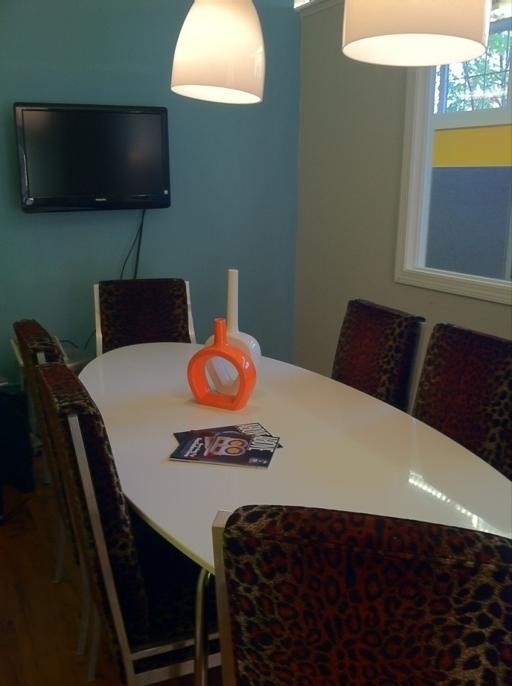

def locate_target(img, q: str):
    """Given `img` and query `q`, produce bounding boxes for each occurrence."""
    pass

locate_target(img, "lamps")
[339,0,489,66]
[171,0,267,106]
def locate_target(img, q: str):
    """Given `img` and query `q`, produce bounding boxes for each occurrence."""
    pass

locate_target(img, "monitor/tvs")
[13,100,172,214]
